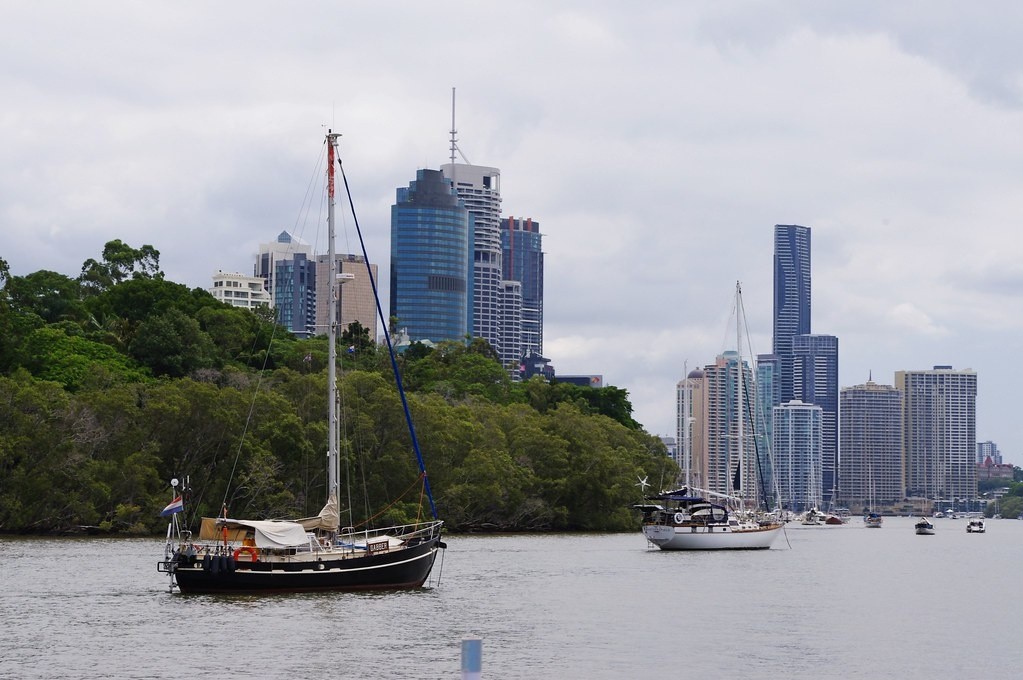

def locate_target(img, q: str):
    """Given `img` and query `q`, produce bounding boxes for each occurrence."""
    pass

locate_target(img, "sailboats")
[156,128,447,595]
[632,279,786,551]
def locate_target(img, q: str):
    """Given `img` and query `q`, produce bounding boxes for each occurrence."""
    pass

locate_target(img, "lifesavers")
[234,547,257,562]
[674,512,684,524]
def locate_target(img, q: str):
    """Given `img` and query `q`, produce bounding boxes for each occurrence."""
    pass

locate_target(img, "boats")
[801,485,851,525]
[966,519,986,533]
[914,517,936,535]
[864,511,883,528]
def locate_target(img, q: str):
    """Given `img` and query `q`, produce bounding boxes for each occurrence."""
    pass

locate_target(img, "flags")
[302,354,312,363]
[346,345,355,354]
[160,495,184,516]
